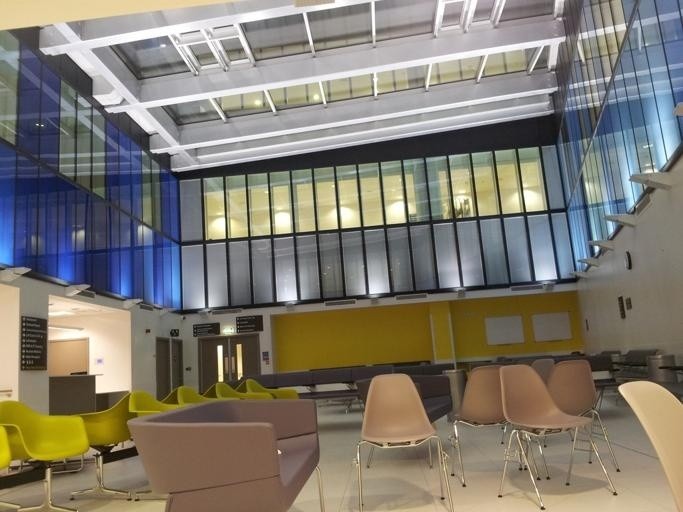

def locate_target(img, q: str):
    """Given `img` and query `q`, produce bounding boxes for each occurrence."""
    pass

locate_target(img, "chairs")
[497,363,620,510]
[349,371,457,512]
[0,399,92,509]
[449,364,529,487]
[70,392,135,503]
[0,347,683,511]
[542,357,620,487]
[125,398,327,511]
[611,376,683,510]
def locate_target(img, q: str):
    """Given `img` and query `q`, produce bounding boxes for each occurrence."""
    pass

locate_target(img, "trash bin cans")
[648,354,678,383]
